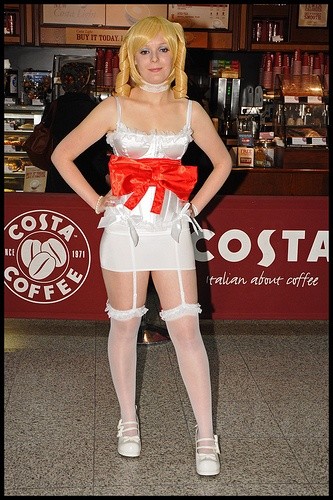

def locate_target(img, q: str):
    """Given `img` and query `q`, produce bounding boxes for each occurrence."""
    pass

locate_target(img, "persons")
[50,17,233,477]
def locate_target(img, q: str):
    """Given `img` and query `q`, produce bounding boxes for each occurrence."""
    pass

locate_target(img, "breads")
[4,124,34,173]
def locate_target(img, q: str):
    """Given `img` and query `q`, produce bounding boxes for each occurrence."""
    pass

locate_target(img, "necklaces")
[138,80,168,93]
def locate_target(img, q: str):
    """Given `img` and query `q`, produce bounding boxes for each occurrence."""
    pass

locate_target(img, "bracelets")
[192,205,199,217]
[95,195,103,215]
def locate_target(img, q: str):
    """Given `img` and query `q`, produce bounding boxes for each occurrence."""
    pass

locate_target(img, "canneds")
[6,13,17,34]
[254,20,280,42]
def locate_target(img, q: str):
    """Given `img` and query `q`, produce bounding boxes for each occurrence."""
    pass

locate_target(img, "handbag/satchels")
[23,100,58,171]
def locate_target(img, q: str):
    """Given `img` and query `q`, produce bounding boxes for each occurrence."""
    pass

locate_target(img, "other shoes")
[195,425,221,476]
[116,405,141,457]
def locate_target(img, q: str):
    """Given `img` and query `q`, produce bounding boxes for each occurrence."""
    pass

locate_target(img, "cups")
[260,49,329,96]
[287,118,294,125]
[296,118,303,125]
[95,49,119,86]
